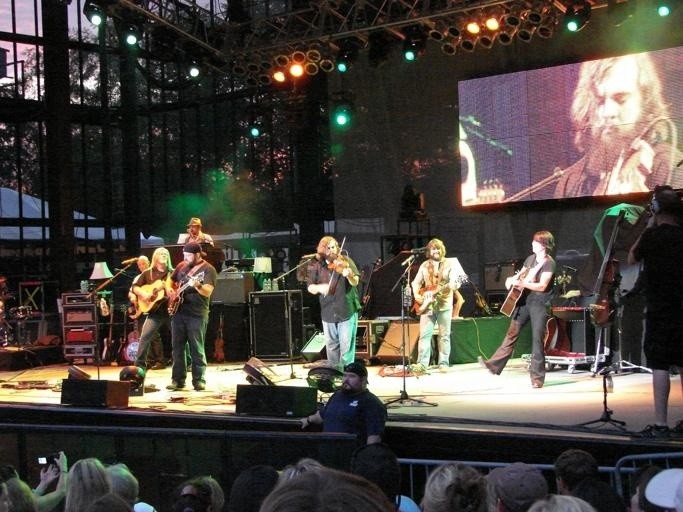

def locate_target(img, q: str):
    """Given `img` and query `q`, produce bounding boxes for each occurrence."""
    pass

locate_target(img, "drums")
[9,306,32,318]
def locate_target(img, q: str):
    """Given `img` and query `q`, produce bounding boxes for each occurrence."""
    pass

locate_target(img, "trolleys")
[541,305,606,373]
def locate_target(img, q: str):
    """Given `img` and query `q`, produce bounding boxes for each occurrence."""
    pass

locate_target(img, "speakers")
[234,382,317,421]
[207,270,254,304]
[58,379,129,409]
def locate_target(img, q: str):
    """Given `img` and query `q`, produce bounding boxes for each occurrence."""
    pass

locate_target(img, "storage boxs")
[18,281,58,314]
[250,292,303,359]
[355,320,390,359]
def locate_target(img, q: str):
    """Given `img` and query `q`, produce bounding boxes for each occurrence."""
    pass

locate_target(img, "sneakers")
[195,383,205,390]
[670,420,683,440]
[438,364,449,374]
[165,381,186,389]
[634,424,669,438]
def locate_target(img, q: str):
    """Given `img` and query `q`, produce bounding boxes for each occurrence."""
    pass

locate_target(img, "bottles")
[273,284,278,291]
[272,279,276,291]
[85,280,88,293]
[268,284,271,292]
[264,284,268,291]
[81,280,86,294]
[263,279,268,291]
[268,278,271,284]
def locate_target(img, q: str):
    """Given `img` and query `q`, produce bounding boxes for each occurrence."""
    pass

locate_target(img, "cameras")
[35,453,61,468]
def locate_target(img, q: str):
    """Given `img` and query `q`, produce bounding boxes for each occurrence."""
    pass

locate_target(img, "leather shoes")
[477,355,495,375]
[532,381,543,388]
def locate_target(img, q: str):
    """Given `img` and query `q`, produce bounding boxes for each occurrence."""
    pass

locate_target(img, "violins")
[592,138,655,198]
[328,254,357,285]
[213,315,225,362]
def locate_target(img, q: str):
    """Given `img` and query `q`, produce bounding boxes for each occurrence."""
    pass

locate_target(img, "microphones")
[121,258,139,265]
[301,254,319,260]
[586,121,620,183]
[410,247,429,254]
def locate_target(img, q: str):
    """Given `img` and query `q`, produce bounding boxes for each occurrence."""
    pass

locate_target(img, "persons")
[129,247,191,372]
[261,473,398,512]
[350,444,421,512]
[184,218,212,245]
[128,255,165,369]
[411,239,468,377]
[66,458,111,512]
[298,363,392,472]
[476,231,559,389]
[626,185,683,438]
[307,237,360,373]
[0,451,67,512]
[549,51,683,198]
[164,243,217,391]
[422,460,488,511]
[554,449,598,496]
[169,476,211,511]
[106,463,155,512]
[630,466,683,512]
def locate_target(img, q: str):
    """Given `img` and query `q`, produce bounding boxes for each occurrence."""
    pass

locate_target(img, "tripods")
[383,256,440,407]
[268,260,308,384]
[576,325,631,431]
[401,255,415,266]
[384,262,431,380]
[598,269,652,375]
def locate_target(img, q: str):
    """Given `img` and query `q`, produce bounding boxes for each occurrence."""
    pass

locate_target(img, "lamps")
[253,256,272,289]
[89,262,114,280]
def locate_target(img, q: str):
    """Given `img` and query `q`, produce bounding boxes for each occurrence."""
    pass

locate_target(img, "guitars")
[125,314,140,361]
[544,318,571,356]
[361,257,381,315]
[168,271,204,315]
[410,274,468,315]
[102,304,117,361]
[118,304,131,363]
[500,267,530,317]
[138,280,168,314]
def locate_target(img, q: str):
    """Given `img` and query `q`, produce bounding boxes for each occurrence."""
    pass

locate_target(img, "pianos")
[141,242,226,274]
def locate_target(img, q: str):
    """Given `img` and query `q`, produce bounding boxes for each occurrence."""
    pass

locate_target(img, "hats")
[350,442,401,496]
[645,467,683,512]
[182,242,208,258]
[186,217,203,228]
[343,362,369,385]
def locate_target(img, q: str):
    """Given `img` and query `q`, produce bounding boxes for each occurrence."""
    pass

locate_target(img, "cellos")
[589,210,625,325]
